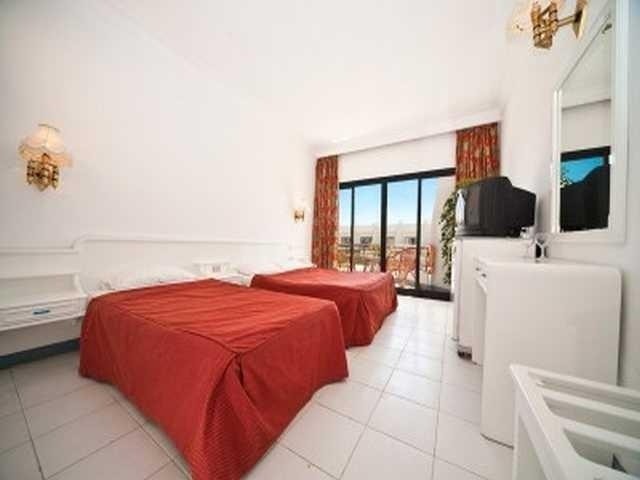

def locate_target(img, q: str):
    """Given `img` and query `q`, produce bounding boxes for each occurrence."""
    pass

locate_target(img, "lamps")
[508,0,591,51]
[15,120,74,192]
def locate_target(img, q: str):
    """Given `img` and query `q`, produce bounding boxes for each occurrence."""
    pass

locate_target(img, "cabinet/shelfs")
[450,235,537,358]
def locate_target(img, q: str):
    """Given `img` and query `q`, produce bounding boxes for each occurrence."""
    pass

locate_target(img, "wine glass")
[534,233,550,262]
[520,226,536,262]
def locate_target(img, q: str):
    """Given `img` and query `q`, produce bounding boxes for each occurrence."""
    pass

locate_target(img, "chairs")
[337,235,434,274]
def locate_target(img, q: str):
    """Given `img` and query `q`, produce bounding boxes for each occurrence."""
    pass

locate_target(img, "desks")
[469,246,623,448]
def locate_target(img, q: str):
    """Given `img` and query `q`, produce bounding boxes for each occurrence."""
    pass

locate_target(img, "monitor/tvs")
[560,164,611,231]
[465,175,536,237]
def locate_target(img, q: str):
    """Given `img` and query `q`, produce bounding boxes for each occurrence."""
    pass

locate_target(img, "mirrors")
[554,10,615,232]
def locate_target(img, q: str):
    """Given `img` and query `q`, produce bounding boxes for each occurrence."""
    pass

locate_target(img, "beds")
[78,278,350,480]
[251,266,399,346]
